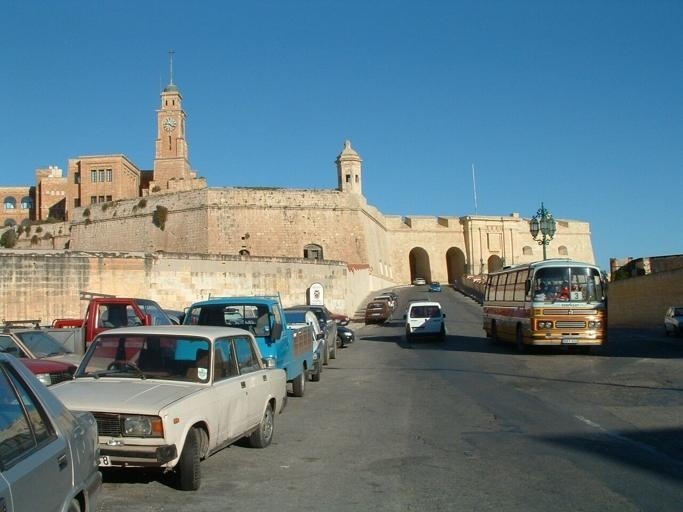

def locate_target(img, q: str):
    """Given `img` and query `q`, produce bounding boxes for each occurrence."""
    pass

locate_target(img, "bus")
[481,257,609,356]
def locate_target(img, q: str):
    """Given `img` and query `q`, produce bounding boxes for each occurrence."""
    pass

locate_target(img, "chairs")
[136,347,226,381]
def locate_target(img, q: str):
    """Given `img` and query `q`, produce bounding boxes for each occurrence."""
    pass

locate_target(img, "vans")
[402,301,446,343]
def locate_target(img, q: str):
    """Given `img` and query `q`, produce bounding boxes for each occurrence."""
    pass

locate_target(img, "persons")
[534,282,582,300]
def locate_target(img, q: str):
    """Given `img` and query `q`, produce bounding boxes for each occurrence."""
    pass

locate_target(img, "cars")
[364,292,399,325]
[413,277,426,285]
[664,306,683,334]
[429,281,442,293]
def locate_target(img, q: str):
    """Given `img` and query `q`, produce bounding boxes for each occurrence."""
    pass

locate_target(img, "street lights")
[528,202,557,261]
